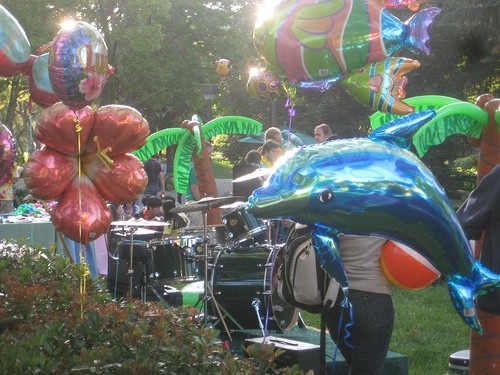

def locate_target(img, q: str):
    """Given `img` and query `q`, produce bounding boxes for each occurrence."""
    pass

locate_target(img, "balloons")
[0,123,17,178]
[246,136,500,336]
[0,5,115,108]
[47,22,109,111]
[23,100,150,243]
[215,0,442,115]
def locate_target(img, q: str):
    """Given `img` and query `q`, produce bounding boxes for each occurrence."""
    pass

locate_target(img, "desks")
[0,218,108,275]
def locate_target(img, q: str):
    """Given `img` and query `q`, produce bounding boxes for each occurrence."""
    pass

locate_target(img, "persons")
[314,124,337,144]
[454,162,500,375]
[322,234,394,375]
[142,155,167,204]
[106,195,205,230]
[12,162,27,210]
[233,127,284,196]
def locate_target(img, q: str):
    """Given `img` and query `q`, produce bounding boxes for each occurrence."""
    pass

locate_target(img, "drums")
[206,245,299,333]
[220,203,267,244]
[116,224,228,287]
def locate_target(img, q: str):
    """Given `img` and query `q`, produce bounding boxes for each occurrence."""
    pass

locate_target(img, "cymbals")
[109,218,172,227]
[109,227,161,236]
[169,195,248,214]
[218,201,245,209]
